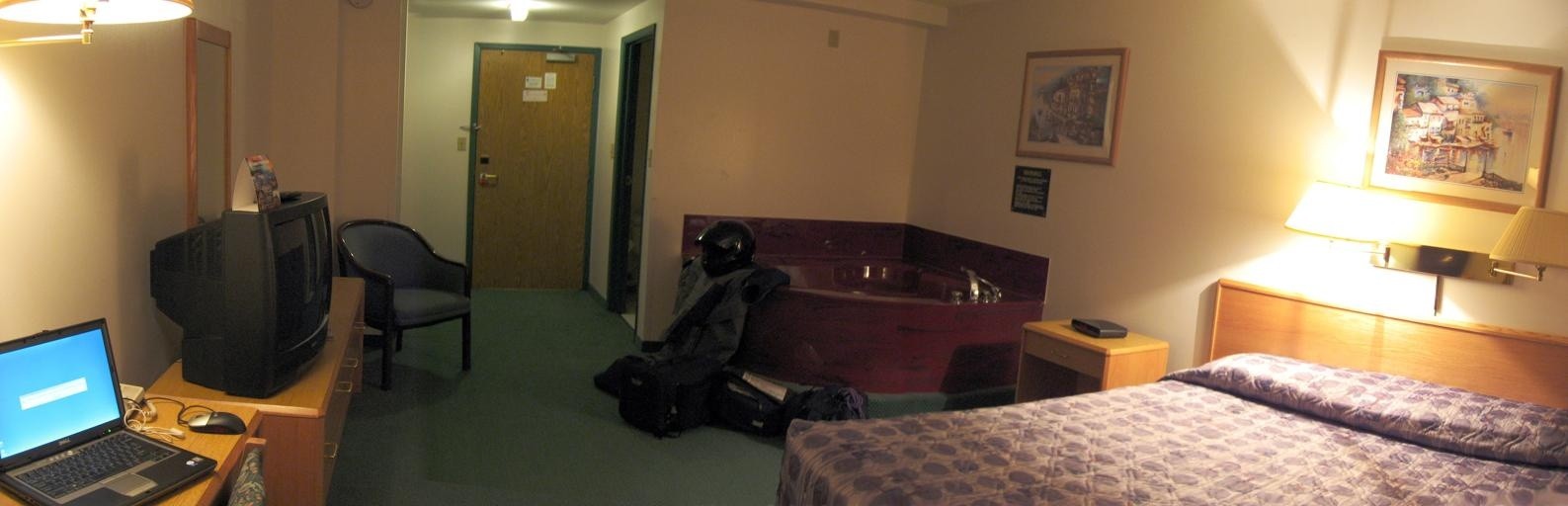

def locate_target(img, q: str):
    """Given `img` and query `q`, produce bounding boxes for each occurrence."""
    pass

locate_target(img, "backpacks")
[715,368,797,437]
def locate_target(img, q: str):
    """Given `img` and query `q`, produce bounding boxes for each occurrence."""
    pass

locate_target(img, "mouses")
[190,412,246,434]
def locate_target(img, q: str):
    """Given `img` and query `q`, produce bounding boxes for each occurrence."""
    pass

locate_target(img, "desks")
[1013,319,1170,403]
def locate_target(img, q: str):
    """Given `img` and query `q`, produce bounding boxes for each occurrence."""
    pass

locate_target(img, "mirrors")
[184,17,234,229]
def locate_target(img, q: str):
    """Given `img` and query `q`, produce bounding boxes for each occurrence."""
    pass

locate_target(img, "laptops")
[0,317,217,506]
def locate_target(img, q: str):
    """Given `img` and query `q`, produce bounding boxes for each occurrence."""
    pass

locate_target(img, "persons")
[592,217,788,438]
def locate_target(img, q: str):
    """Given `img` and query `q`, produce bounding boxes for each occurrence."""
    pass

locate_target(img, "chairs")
[336,219,473,391]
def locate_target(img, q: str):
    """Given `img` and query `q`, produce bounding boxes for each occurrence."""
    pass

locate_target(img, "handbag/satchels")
[779,372,868,437]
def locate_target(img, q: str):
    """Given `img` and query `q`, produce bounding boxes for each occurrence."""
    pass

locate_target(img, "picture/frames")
[1361,49,1563,216]
[1014,46,1130,167]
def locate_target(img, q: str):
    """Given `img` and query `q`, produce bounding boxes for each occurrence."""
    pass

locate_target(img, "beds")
[775,279,1568,506]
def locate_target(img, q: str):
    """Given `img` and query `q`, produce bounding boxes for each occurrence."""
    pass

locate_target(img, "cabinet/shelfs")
[144,277,366,506]
[1,398,265,506]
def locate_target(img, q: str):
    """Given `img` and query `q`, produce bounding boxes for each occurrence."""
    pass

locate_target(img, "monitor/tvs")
[159,191,333,398]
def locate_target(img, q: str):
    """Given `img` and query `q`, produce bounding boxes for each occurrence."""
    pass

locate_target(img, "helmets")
[694,220,754,267]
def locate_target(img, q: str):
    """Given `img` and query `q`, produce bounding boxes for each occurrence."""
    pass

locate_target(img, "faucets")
[979,278,1001,301]
[960,265,980,301]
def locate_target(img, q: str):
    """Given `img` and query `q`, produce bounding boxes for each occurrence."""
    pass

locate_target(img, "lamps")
[0,0,194,47]
[1488,207,1568,281]
[1284,179,1385,245]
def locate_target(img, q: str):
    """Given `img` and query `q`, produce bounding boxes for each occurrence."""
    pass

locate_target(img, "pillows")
[1164,353,1568,467]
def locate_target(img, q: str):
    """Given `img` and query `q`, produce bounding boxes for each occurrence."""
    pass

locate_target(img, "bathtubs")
[758,253,1045,391]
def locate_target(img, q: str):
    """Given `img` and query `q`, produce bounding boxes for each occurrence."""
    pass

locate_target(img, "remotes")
[280,191,301,201]
